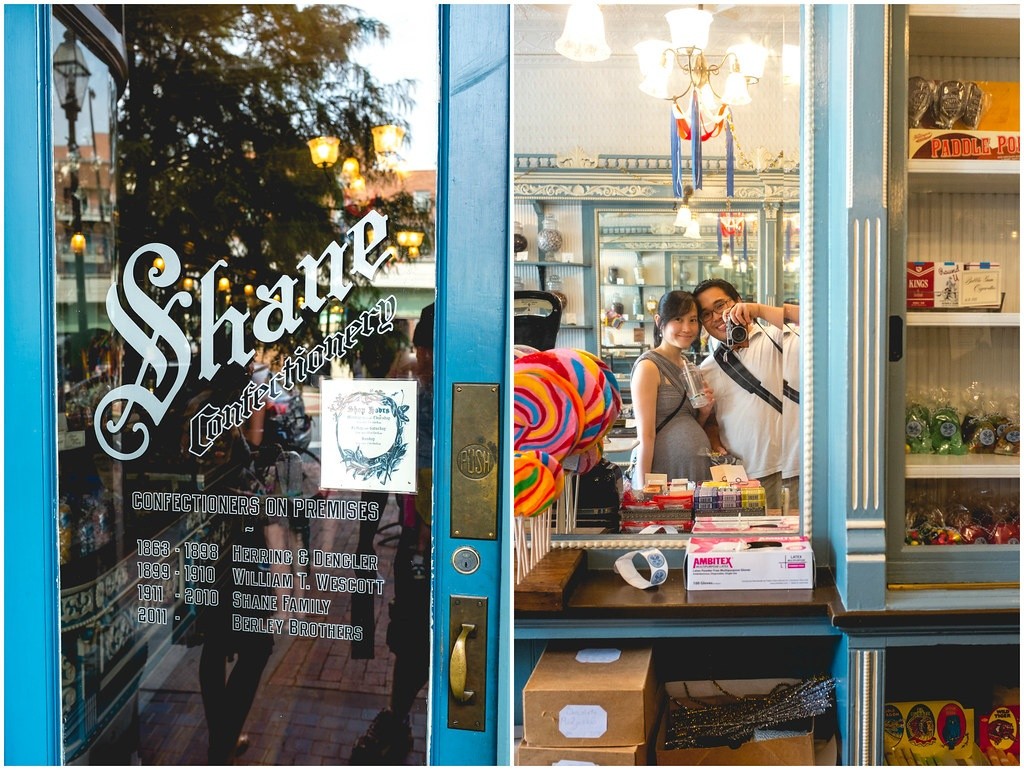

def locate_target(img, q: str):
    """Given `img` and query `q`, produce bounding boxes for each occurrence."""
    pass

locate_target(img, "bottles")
[252,362,288,402]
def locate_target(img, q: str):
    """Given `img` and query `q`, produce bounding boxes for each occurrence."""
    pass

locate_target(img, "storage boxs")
[681,534,817,591]
[617,479,800,532]
[906,126,1020,162]
[515,641,839,766]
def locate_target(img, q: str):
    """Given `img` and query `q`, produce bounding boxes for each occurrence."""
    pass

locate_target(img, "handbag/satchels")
[351,545,376,659]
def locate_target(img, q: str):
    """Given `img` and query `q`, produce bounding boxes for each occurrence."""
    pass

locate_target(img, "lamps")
[303,122,424,263]
[555,4,613,62]
[718,243,747,274]
[67,230,258,299]
[632,4,771,125]
[673,186,703,240]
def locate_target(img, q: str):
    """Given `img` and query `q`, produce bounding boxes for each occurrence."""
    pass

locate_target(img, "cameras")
[726,316,749,349]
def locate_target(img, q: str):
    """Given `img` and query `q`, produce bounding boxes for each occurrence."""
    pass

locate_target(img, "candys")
[512,348,625,523]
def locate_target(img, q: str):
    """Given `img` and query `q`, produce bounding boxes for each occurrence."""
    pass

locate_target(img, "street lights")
[51,29,95,452]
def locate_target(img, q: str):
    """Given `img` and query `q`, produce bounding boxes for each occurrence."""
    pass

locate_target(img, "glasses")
[698,297,734,324]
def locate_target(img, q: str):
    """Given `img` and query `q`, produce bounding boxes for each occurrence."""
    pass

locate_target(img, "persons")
[628,290,715,492]
[783,301,802,510]
[694,277,783,510]
[198,302,437,767]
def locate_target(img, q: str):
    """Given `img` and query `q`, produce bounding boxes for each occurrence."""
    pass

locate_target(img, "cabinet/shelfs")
[513,260,595,329]
[885,4,1020,586]
[600,282,698,323]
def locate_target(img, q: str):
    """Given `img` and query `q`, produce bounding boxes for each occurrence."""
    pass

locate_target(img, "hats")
[412,304,434,344]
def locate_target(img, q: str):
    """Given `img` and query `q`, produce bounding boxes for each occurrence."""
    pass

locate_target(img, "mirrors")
[593,208,801,393]
[510,4,817,554]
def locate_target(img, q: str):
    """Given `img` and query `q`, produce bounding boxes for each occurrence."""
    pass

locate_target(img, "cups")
[678,363,707,409]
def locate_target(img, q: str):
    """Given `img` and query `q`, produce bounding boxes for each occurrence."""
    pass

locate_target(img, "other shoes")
[209,734,260,766]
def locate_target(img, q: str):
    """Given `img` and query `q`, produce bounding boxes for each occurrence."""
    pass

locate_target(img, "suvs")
[148,361,313,460]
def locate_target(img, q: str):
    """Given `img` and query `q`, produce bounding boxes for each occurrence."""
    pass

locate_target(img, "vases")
[513,221,528,262]
[546,274,568,327]
[536,214,563,262]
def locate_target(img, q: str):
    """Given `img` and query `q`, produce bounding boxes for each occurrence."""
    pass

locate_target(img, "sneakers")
[350,707,412,767]
